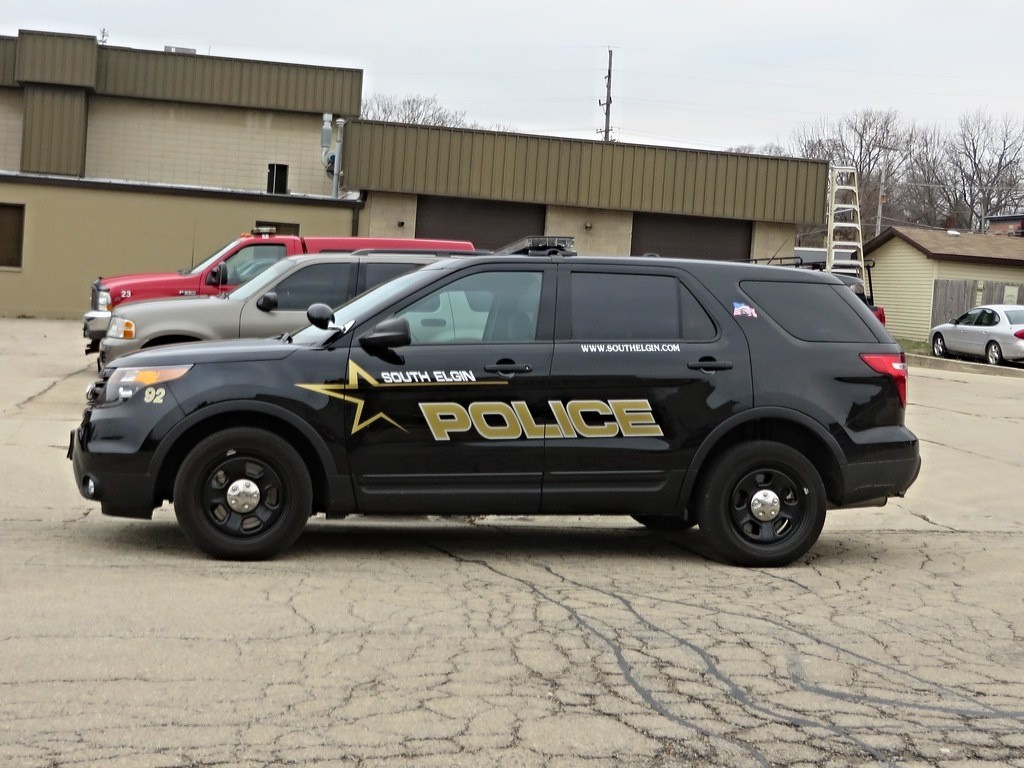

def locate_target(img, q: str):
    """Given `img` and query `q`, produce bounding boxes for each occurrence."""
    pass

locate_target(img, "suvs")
[65,222,923,570]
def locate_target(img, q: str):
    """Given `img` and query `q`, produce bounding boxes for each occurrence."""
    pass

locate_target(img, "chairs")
[506,309,531,341]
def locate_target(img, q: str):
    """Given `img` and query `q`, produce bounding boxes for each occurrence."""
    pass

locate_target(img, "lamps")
[584,222,593,232]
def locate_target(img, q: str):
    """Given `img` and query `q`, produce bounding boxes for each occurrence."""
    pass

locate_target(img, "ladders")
[825,165,864,289]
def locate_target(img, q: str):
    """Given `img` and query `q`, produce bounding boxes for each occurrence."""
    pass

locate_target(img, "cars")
[928,303,1024,367]
